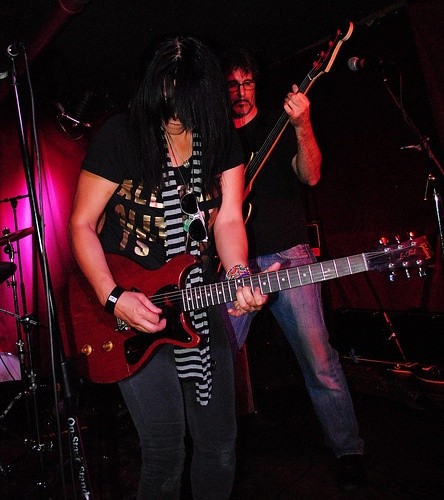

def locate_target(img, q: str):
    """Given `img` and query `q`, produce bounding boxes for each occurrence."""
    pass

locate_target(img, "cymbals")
[0,261,18,284]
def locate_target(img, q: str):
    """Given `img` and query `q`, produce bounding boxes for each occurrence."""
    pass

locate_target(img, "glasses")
[180,193,208,242]
[228,80,256,91]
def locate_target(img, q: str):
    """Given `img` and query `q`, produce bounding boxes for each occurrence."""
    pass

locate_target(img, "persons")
[66,36,269,500]
[211,47,365,492]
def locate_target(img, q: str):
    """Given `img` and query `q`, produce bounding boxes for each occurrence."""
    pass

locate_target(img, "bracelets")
[225,264,252,280]
[103,286,127,315]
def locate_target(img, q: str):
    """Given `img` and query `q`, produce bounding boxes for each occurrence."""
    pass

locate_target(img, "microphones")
[0,41,23,79]
[348,57,385,71]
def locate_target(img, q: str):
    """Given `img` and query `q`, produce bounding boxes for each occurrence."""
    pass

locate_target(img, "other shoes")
[336,454,369,490]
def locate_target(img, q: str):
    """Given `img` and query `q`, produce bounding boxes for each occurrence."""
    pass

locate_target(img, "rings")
[249,306,255,313]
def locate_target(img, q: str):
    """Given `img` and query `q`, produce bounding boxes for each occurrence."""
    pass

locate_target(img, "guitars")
[50,233,433,385]
[197,18,357,278]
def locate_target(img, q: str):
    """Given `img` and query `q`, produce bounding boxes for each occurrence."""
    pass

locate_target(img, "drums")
[0,351,24,382]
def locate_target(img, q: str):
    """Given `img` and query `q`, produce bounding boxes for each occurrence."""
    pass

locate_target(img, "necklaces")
[168,133,196,192]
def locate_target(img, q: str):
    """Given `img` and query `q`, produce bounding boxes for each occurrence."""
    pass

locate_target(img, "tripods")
[0,201,72,477]
